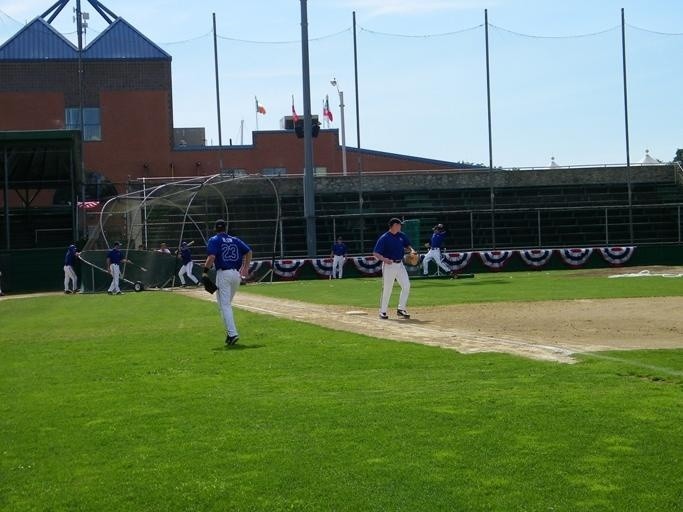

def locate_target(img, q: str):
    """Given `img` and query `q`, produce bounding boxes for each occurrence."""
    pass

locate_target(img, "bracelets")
[204,266,209,273]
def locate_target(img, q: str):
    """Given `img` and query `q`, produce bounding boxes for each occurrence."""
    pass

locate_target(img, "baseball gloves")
[404,251,418,266]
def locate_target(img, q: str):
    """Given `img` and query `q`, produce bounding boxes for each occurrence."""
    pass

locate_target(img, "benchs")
[142,183,683,266]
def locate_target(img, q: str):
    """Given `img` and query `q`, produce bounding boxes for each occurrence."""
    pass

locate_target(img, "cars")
[101,238,129,249]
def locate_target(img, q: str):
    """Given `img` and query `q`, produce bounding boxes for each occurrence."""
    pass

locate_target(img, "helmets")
[182,242,187,249]
[69,245,76,253]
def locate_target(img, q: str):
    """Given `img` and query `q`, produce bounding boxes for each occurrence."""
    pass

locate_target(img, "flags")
[256,99,265,114]
[292,97,299,122]
[323,97,333,123]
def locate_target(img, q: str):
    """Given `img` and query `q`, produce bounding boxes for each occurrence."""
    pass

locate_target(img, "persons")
[138,244,144,248]
[329,237,348,278]
[178,242,201,289]
[202,220,252,346]
[63,244,82,294]
[107,241,132,295]
[373,218,417,319]
[422,225,456,277]
[157,243,170,254]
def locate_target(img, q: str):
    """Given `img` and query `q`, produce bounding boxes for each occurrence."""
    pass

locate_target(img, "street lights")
[330,79,348,176]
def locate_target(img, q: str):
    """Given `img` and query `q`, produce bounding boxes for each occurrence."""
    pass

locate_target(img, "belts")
[391,260,402,263]
[216,267,240,270]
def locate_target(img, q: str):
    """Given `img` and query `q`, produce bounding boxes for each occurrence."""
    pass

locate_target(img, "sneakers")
[224,335,239,348]
[106,290,112,295]
[66,290,72,294]
[179,284,186,288]
[196,282,202,288]
[396,308,411,319]
[115,292,124,295]
[379,311,389,319]
[73,289,80,293]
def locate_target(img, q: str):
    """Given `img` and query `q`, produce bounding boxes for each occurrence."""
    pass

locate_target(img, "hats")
[432,225,442,231]
[214,219,227,230]
[160,243,166,246]
[388,218,407,226]
[113,241,122,246]
